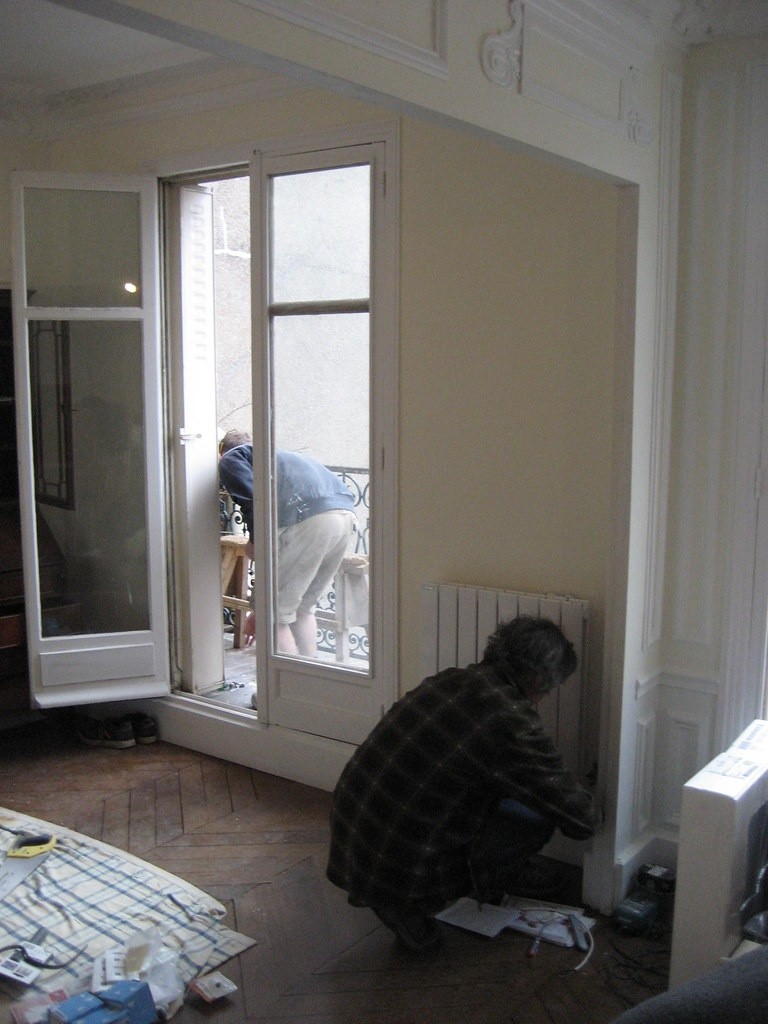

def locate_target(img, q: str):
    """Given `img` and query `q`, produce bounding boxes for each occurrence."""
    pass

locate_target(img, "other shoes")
[122,711,159,744]
[348,877,378,908]
[251,691,260,708]
[364,884,442,948]
[77,716,136,749]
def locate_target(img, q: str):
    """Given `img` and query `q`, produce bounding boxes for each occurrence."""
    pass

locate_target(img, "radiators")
[420,581,593,793]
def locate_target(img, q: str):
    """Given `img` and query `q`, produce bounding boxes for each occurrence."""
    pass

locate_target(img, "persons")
[327,615,604,958]
[220,430,357,709]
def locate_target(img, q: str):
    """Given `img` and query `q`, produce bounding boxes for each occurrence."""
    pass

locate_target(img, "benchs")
[220,535,369,663]
